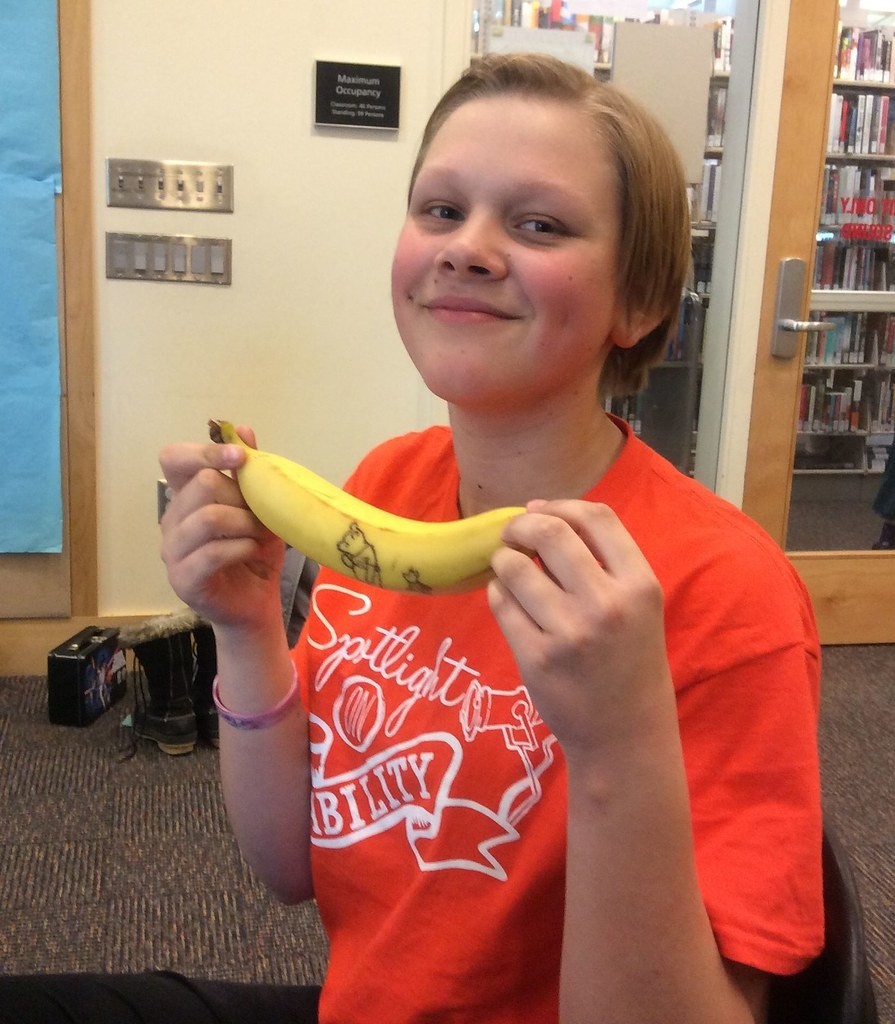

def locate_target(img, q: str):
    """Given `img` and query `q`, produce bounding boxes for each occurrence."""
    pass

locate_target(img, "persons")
[159,52,827,1024]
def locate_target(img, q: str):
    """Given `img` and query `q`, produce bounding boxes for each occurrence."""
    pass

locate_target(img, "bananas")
[217,420,517,593]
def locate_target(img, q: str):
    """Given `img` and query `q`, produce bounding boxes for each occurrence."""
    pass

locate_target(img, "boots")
[119,610,222,756]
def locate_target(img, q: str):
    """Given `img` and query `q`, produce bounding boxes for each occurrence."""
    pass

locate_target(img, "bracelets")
[212,659,304,729]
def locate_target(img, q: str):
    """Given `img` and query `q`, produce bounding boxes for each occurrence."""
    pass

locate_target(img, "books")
[476,0,894,471]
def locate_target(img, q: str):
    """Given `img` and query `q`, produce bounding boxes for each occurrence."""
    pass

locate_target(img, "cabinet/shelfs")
[471,51,895,475]
